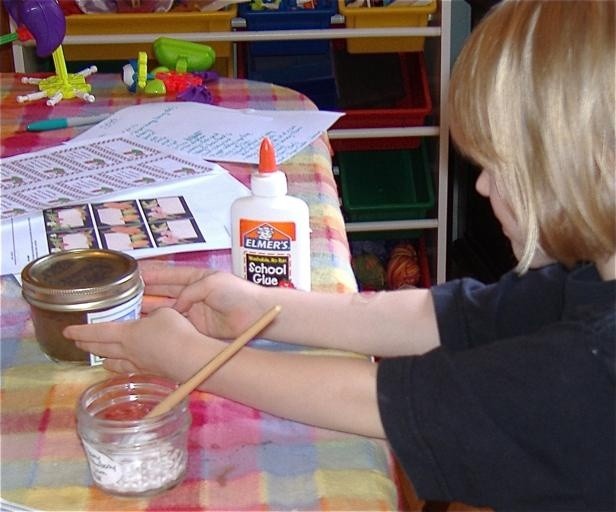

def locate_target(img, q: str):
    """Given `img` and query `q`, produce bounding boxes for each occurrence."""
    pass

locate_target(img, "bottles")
[228,136,313,291]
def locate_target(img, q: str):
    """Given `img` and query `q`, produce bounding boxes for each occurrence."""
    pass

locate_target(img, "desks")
[0,73,406,510]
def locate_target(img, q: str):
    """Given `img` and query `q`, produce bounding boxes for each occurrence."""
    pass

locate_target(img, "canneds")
[21,248,146,365]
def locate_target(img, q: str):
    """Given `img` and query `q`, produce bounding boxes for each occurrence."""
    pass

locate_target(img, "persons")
[60,0,615,510]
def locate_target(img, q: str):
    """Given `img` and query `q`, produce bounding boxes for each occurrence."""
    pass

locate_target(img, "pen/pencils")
[27,112,111,132]
[121,306,283,447]
